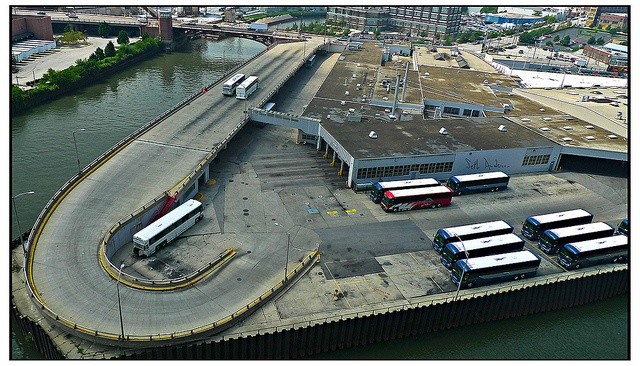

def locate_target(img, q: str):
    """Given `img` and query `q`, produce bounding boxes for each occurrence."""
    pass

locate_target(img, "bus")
[133,198,203,257]
[257,103,275,126]
[537,220,614,254]
[307,54,316,66]
[370,177,441,204]
[447,170,509,196]
[450,249,541,289]
[557,234,628,270]
[521,207,593,241]
[432,219,514,254]
[236,75,258,100]
[221,72,245,96]
[440,232,525,270]
[380,185,454,213]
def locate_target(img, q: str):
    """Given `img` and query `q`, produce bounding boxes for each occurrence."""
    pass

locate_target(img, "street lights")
[73,128,86,173]
[117,263,125,339]
[12,192,34,252]
[274,222,290,280]
[455,233,468,300]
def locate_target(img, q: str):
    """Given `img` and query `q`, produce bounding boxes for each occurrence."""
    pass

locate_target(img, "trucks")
[247,23,268,30]
